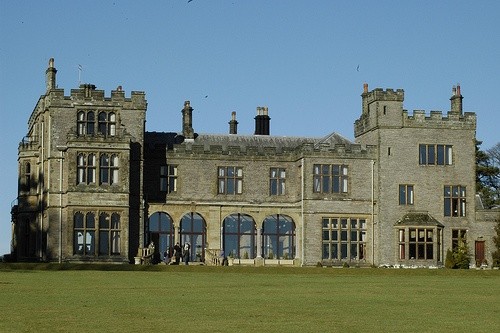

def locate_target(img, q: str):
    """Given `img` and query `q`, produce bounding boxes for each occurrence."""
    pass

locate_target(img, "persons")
[163,242,191,266]
[148,241,155,259]
